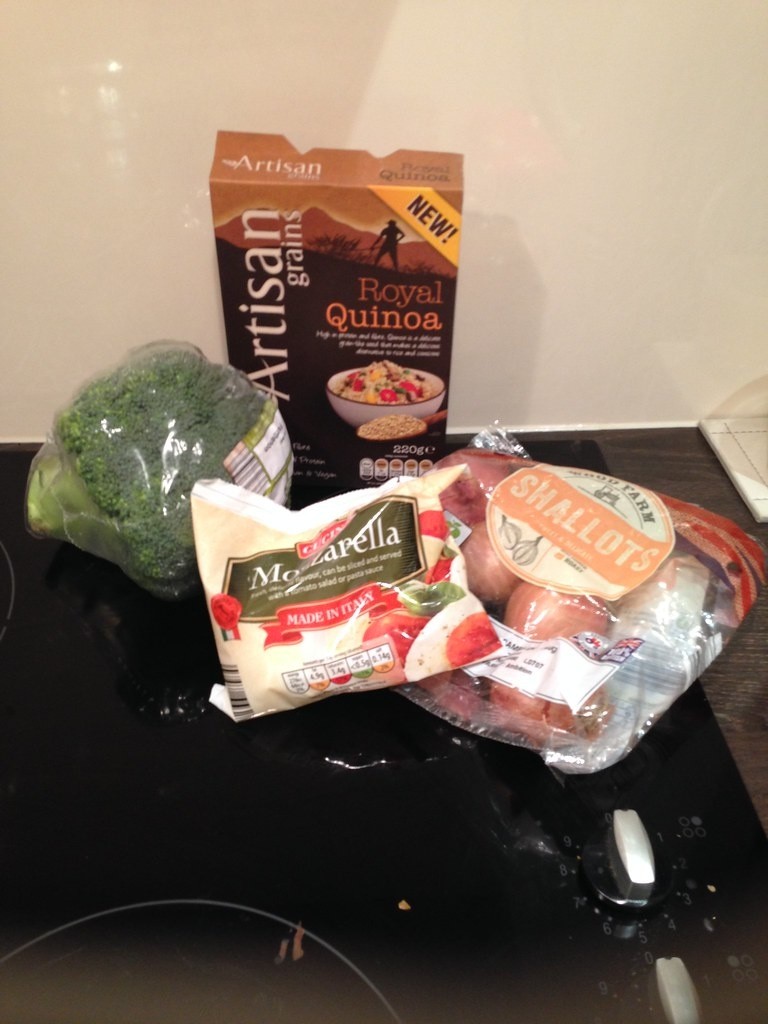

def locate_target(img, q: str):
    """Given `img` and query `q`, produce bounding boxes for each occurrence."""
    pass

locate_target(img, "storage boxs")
[213,130,463,492]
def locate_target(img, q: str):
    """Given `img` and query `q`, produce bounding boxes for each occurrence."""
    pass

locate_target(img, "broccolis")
[27,348,278,596]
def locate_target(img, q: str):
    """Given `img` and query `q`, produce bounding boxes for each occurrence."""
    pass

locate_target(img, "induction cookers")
[0,440,768,1024]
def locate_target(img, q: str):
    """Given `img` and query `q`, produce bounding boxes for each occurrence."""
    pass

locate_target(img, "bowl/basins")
[325,366,447,428]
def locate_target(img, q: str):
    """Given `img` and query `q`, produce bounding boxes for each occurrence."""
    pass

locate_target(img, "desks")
[0,425,768,1024]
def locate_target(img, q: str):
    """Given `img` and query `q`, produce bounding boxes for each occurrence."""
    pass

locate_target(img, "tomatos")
[347,371,417,402]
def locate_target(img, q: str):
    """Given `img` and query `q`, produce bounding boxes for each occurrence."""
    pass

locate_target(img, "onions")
[373,450,613,742]
